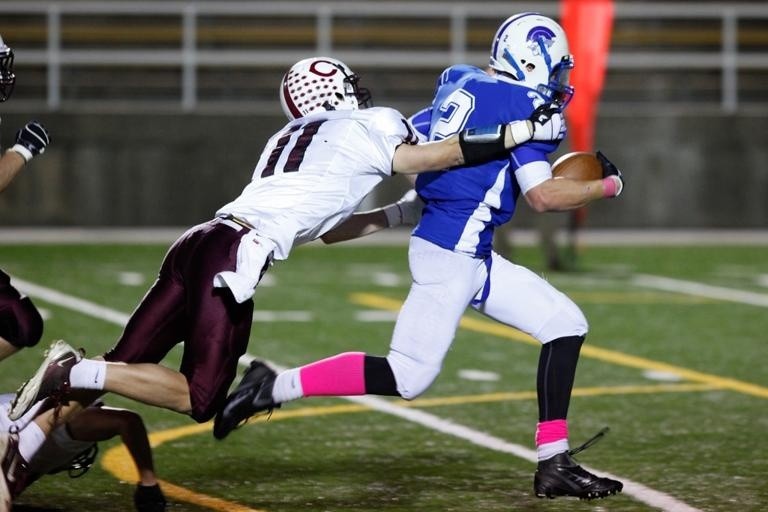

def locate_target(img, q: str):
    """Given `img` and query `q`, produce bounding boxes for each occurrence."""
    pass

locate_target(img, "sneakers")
[532,450,624,503]
[212,358,284,442]
[7,337,87,422]
[0,430,30,512]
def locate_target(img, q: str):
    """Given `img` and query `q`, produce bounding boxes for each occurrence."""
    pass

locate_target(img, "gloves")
[132,481,168,512]
[528,101,568,145]
[13,119,52,159]
[594,149,626,198]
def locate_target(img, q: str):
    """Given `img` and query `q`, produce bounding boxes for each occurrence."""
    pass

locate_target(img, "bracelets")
[508,119,531,144]
[602,178,616,199]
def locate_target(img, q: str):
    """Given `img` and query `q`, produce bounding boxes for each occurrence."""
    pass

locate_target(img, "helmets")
[278,55,375,123]
[0,32,18,103]
[488,11,576,114]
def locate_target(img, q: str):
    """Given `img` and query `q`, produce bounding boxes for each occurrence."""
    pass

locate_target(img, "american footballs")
[551,149,604,181]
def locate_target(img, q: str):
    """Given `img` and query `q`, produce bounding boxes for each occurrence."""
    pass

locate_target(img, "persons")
[0,34,50,361]
[214,12,624,501]
[0,392,167,512]
[0,56,568,512]
